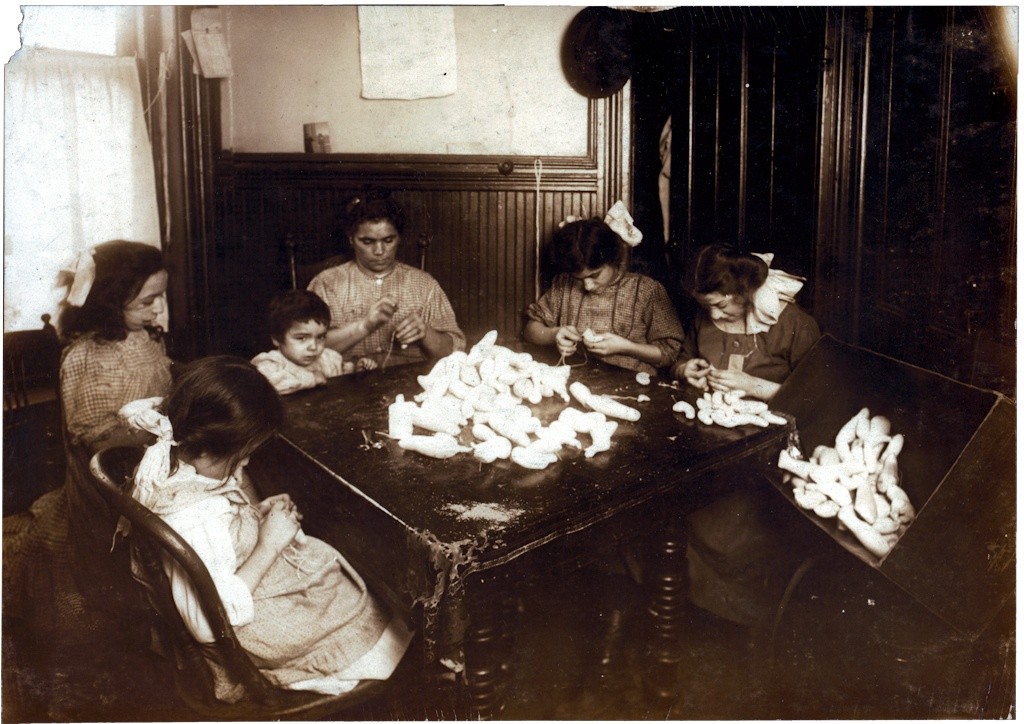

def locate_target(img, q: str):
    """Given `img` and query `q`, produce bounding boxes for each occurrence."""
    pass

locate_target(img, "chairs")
[3,314,64,512]
[85,444,408,722]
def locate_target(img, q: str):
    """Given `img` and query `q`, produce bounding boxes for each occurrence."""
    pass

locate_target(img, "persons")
[670,242,820,624]
[56,239,175,450]
[248,290,376,395]
[121,354,413,696]
[523,217,685,377]
[307,185,466,371]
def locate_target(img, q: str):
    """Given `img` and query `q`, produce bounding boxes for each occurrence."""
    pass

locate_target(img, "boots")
[582,608,631,693]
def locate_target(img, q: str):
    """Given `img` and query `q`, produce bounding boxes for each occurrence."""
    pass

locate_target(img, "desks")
[268,339,797,722]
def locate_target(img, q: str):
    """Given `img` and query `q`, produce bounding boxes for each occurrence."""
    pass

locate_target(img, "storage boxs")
[760,335,1017,642]
